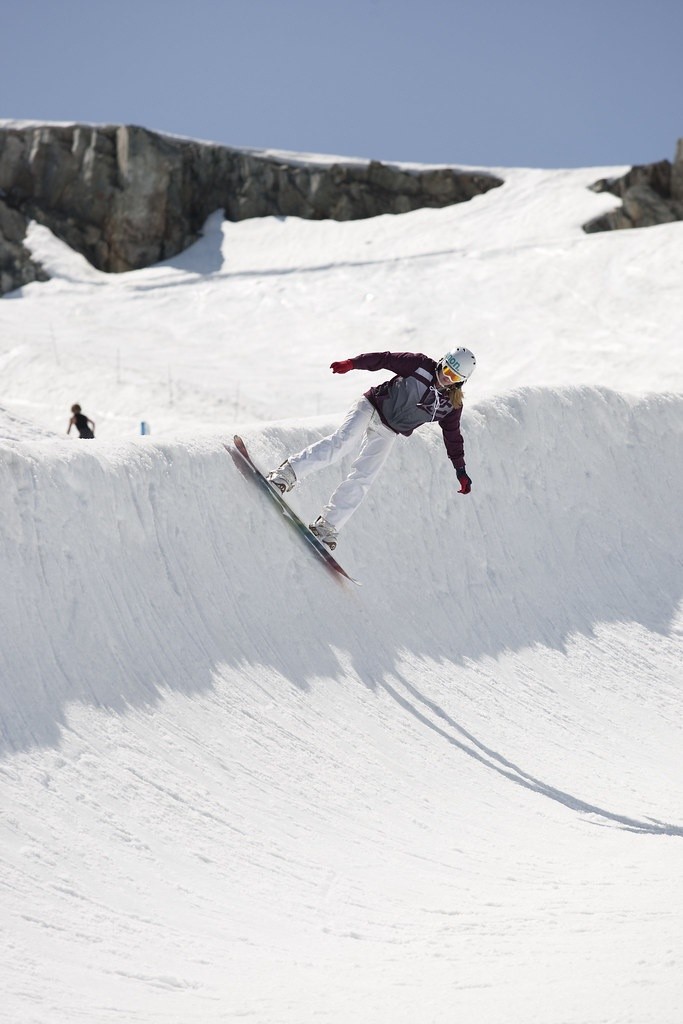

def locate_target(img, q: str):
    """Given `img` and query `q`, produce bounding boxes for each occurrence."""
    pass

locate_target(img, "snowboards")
[233,429,365,592]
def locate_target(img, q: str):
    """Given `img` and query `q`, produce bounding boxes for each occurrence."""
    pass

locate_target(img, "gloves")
[330,359,353,374]
[457,467,472,494]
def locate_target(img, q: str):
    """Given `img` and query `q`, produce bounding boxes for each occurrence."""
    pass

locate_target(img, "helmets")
[444,346,476,378]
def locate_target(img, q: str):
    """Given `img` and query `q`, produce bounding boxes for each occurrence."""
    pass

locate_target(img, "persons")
[266,345,477,551]
[67,404,95,439]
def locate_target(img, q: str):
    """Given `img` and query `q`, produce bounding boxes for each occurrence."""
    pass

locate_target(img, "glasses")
[442,359,467,382]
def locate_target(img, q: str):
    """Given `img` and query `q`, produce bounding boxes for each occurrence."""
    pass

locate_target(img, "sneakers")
[309,518,340,551]
[267,466,296,496]
